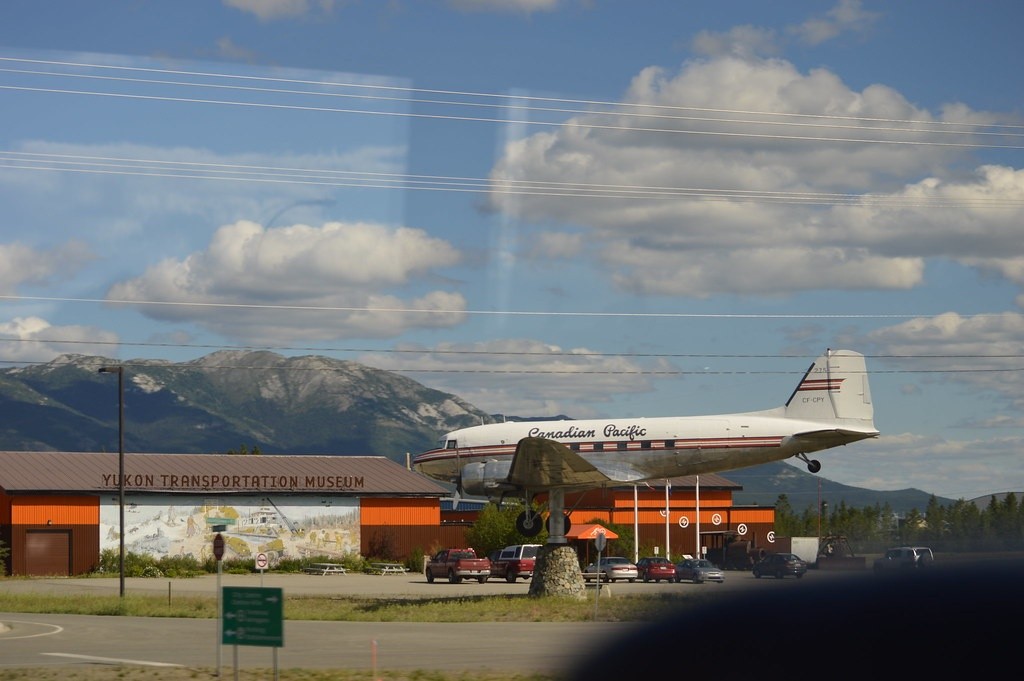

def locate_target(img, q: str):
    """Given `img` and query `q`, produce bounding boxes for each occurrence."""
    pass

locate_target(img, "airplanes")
[413,348,883,539]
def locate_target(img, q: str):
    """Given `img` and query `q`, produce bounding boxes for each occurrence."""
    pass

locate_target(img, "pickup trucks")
[426,548,491,585]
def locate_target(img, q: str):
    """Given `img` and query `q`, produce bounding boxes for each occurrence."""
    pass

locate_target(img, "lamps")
[48,520,51,525]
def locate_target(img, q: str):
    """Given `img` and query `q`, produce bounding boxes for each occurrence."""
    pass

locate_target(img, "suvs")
[874,547,935,570]
[490,544,542,584]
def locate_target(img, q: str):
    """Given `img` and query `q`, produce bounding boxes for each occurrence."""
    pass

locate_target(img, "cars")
[754,553,808,581]
[636,558,678,582]
[582,556,638,584]
[678,559,726,583]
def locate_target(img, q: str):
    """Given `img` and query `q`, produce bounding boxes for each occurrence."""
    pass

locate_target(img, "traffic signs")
[217,584,286,649]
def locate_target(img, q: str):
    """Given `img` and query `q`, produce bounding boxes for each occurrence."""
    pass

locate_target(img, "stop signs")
[254,552,270,569]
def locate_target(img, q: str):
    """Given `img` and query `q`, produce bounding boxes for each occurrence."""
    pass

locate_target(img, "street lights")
[98,366,125,598]
